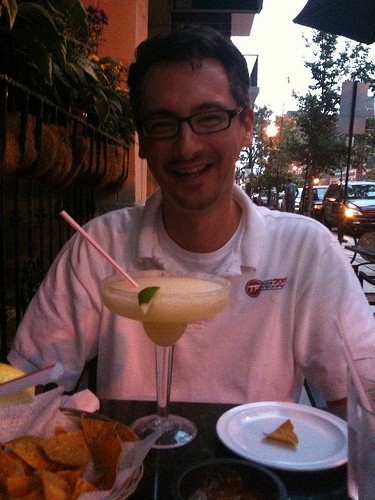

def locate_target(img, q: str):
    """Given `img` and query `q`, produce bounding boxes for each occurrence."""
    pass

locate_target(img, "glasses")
[136,105,245,138]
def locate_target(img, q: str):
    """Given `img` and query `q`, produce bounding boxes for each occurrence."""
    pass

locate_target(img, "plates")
[216,401,348,471]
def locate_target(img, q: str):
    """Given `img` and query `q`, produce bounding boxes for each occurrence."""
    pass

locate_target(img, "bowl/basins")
[179,459,288,500]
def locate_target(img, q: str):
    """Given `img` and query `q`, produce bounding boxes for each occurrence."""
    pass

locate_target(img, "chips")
[262,418,298,448]
[0,419,139,500]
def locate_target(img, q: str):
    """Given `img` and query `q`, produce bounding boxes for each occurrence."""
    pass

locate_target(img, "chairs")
[357,271,375,308]
[350,230,375,265]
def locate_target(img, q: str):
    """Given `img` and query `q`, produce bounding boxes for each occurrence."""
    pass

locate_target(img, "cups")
[345,357,375,500]
[357,388,375,500]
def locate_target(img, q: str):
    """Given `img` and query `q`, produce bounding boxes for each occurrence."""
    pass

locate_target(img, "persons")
[285,179,299,213]
[0,20,375,500]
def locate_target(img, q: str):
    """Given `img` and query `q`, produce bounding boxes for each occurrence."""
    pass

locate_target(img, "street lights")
[265,122,279,207]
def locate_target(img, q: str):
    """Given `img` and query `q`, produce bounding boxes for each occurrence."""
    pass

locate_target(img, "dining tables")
[95,400,348,500]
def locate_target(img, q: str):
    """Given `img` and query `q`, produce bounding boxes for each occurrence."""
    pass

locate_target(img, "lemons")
[138,286,160,313]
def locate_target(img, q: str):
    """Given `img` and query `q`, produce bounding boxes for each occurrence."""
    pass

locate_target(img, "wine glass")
[100,269,230,449]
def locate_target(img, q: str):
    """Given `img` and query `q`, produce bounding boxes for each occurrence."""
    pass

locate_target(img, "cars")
[320,180,375,238]
[250,190,277,206]
[272,187,304,213]
[298,185,330,220]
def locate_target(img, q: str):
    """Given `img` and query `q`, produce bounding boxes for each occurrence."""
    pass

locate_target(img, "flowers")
[0,0,136,145]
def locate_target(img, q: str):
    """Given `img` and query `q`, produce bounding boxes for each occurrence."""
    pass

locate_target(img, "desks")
[346,245,375,260]
